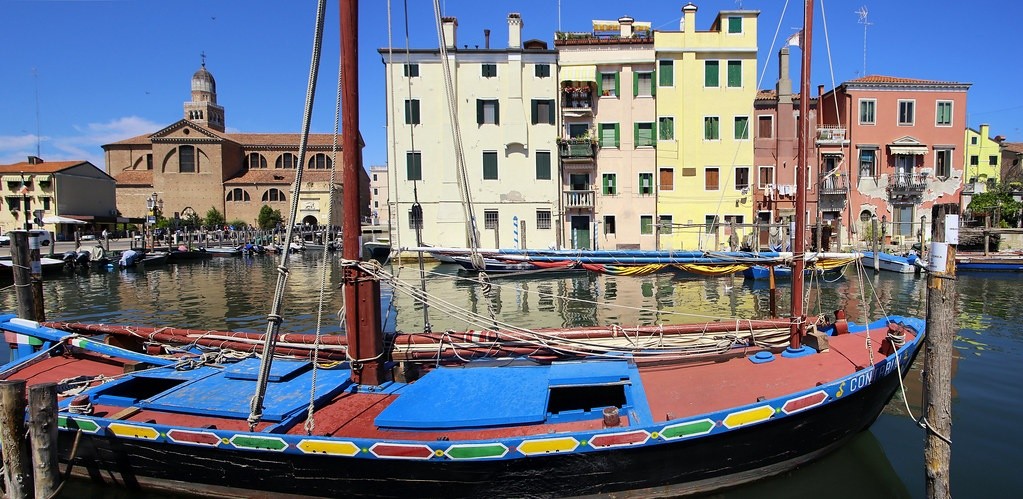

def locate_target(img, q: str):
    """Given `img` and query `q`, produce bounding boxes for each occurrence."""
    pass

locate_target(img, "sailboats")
[0,1,929,499]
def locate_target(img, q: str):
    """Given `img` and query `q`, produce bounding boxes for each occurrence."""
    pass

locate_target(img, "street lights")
[146,192,163,246]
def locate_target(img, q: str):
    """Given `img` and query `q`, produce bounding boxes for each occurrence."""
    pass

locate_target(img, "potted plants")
[603,89,610,96]
[554,30,653,45]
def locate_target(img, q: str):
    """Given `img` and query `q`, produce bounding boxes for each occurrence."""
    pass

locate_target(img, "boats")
[851,243,929,273]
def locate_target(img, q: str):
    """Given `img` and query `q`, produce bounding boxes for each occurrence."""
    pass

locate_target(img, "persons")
[74,227,82,250]
[102,228,108,241]
[157,224,253,242]
[305,220,310,232]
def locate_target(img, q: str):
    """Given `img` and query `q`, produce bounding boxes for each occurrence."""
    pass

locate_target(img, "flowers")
[564,85,591,93]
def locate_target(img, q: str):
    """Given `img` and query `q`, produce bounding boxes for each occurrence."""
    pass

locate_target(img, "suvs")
[29,229,51,246]
[0,229,27,247]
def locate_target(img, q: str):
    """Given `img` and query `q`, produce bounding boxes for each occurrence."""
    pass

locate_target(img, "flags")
[785,30,803,49]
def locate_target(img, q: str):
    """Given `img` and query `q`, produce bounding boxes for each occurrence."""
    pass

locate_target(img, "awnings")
[27,215,92,234]
[560,65,597,84]
[887,144,929,156]
